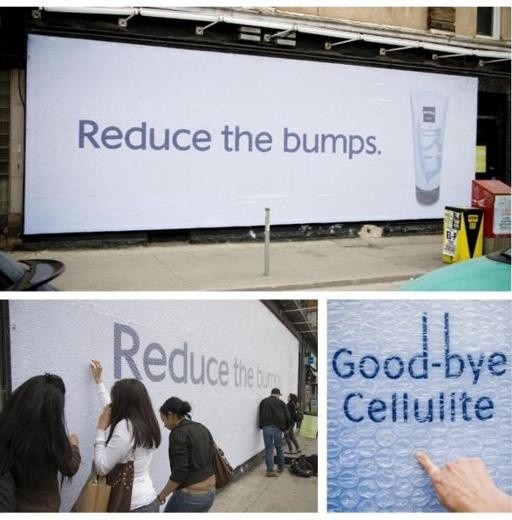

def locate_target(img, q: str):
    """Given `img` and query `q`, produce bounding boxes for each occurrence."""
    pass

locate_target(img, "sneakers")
[265,449,301,478]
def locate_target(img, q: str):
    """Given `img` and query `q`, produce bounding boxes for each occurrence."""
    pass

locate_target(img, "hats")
[270,388,283,396]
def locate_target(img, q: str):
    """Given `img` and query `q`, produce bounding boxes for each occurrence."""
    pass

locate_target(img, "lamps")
[264,24,298,42]
[380,42,424,55]
[119,9,143,27]
[196,16,225,35]
[432,51,480,60]
[325,35,364,49]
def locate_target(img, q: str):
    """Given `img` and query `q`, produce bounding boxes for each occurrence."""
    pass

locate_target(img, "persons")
[88,357,160,512]
[413,450,511,512]
[1,373,81,512]
[282,394,302,454]
[156,396,217,513]
[258,387,291,478]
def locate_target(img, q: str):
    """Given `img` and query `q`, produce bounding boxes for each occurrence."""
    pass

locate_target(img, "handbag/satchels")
[69,455,136,513]
[292,403,304,424]
[207,444,234,488]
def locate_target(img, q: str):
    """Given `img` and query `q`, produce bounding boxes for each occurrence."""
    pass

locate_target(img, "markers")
[90,360,96,369]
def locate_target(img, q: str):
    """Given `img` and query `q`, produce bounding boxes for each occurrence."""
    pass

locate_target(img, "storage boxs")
[471,180,511,238]
[483,237,511,256]
[442,206,485,264]
